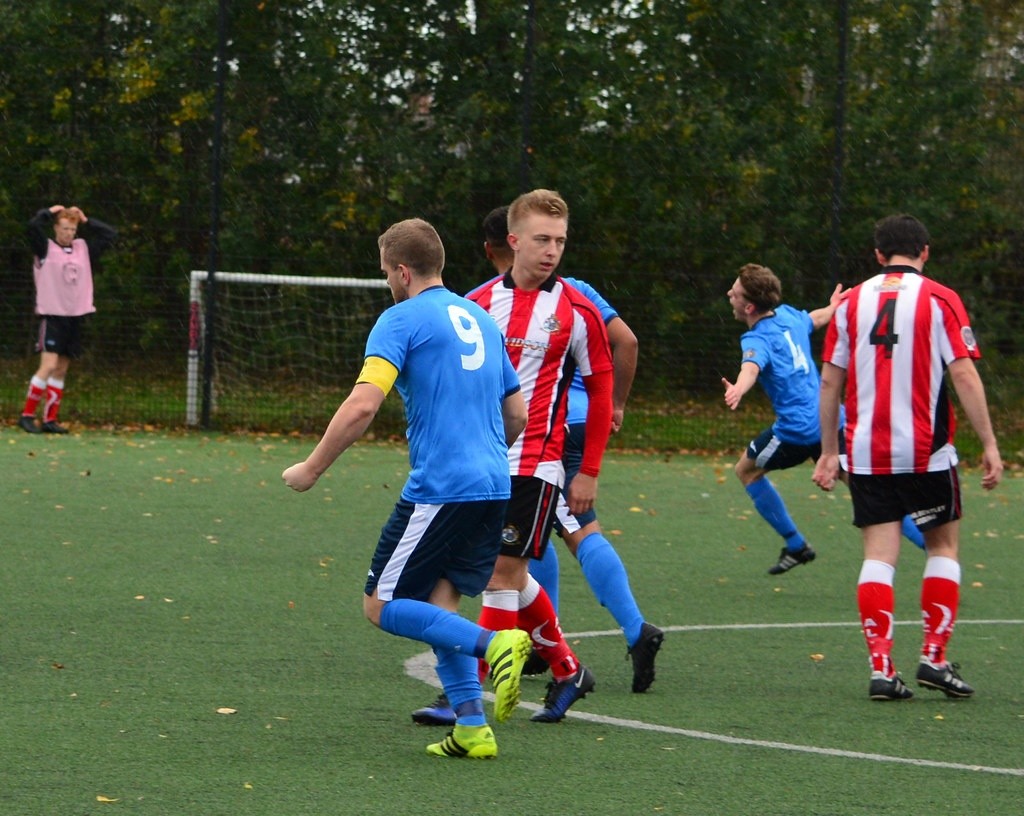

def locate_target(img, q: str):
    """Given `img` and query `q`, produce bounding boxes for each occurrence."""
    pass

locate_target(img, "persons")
[721,263,927,579]
[411,189,613,727]
[282,219,528,763]
[465,201,667,697]
[19,204,114,434]
[816,214,1001,700]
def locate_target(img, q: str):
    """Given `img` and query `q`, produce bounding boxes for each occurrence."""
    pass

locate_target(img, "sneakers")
[409,694,458,727]
[17,412,42,434]
[630,622,664,693]
[425,726,496,759]
[767,539,816,576]
[867,674,915,699]
[486,628,532,722]
[42,422,67,433]
[916,654,975,698]
[532,662,597,724]
